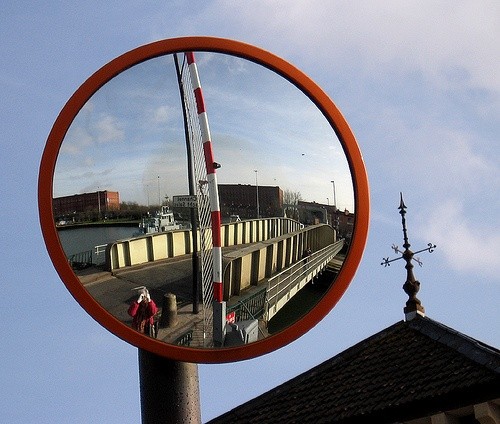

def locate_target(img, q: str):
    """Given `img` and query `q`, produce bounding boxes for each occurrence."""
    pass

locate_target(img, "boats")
[133,203,183,238]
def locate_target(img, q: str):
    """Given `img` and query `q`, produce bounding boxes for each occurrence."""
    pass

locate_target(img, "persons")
[129,286,162,339]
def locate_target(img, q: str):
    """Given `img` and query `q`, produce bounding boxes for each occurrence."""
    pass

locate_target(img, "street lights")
[331,180,338,223]
[254,169,260,219]
